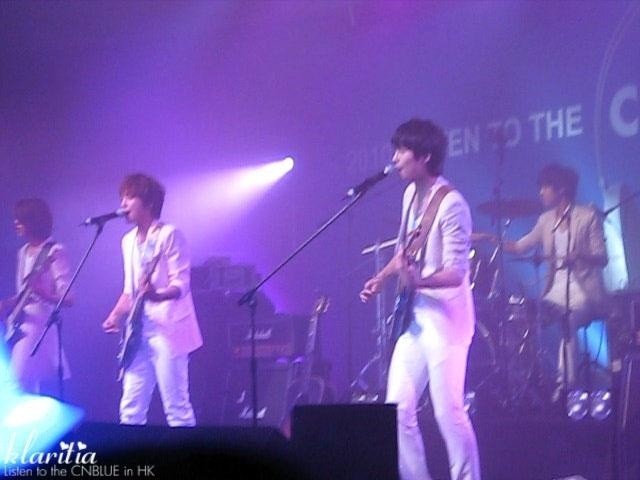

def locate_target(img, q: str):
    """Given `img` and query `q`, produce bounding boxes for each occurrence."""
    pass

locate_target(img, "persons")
[470,163,609,402]
[0,200,75,395]
[102,174,203,426]
[359,118,481,480]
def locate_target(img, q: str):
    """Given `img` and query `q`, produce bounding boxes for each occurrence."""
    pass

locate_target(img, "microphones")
[78,208,125,227]
[551,201,573,233]
[343,163,394,199]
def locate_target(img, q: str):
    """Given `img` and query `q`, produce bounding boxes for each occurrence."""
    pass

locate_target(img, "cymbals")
[477,199,543,219]
[506,252,565,263]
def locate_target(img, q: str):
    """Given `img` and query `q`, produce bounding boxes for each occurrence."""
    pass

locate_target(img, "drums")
[475,292,532,369]
[470,233,505,297]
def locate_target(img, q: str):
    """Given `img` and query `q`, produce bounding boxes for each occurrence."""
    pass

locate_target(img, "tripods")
[507,265,558,416]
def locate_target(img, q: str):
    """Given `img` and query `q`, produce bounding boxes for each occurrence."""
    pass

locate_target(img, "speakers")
[290,403,399,480]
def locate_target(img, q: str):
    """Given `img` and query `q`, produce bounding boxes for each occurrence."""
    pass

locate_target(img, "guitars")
[384,228,420,340]
[1,244,66,345]
[279,296,330,437]
[114,243,163,383]
